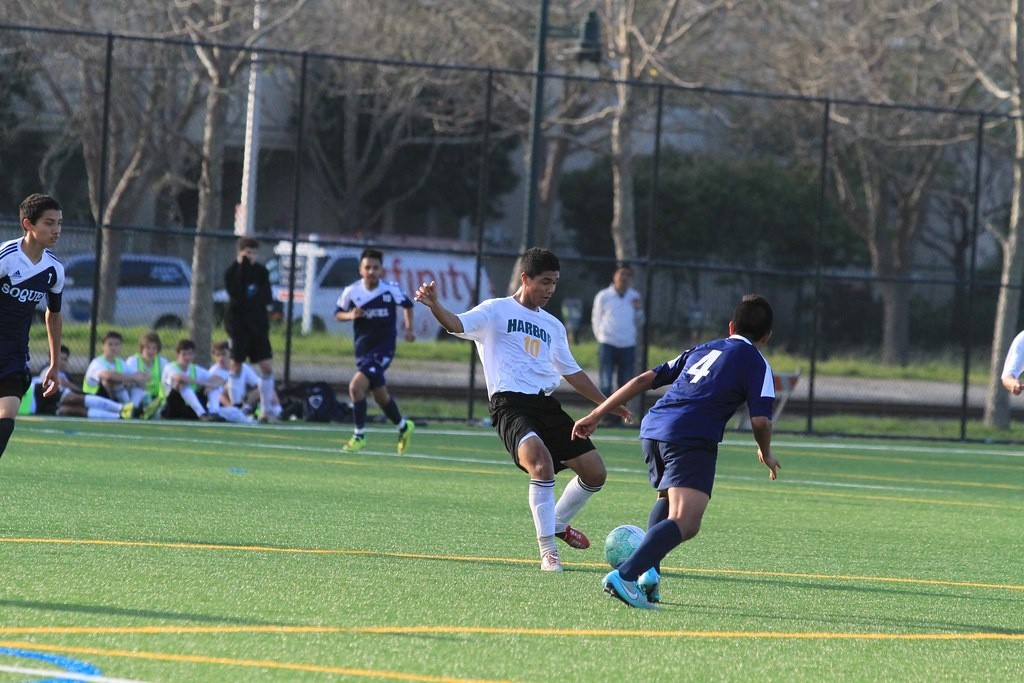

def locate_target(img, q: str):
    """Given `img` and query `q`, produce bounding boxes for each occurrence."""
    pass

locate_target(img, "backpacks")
[303,382,335,423]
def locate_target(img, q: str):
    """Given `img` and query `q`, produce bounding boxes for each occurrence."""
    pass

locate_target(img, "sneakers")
[601,567,660,609]
[541,553,563,573]
[397,420,415,456]
[342,436,367,452]
[121,397,162,420]
[245,411,296,425]
[200,413,225,422]
[555,525,590,550]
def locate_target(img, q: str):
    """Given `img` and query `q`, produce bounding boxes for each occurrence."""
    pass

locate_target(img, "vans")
[37,255,193,332]
[211,234,497,347]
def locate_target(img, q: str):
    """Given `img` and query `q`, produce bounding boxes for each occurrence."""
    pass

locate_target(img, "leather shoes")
[596,416,615,428]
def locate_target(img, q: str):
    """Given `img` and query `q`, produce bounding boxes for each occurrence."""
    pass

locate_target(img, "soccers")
[603,524,647,570]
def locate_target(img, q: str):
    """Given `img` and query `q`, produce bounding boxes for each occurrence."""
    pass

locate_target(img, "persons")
[209,341,298,422]
[590,260,646,426]
[40,344,134,421]
[81,331,162,420]
[412,248,634,569]
[124,333,170,419]
[571,294,782,610]
[0,194,65,455]
[158,338,227,423]
[333,249,416,456]
[1001,331,1024,397]
[224,237,276,424]
